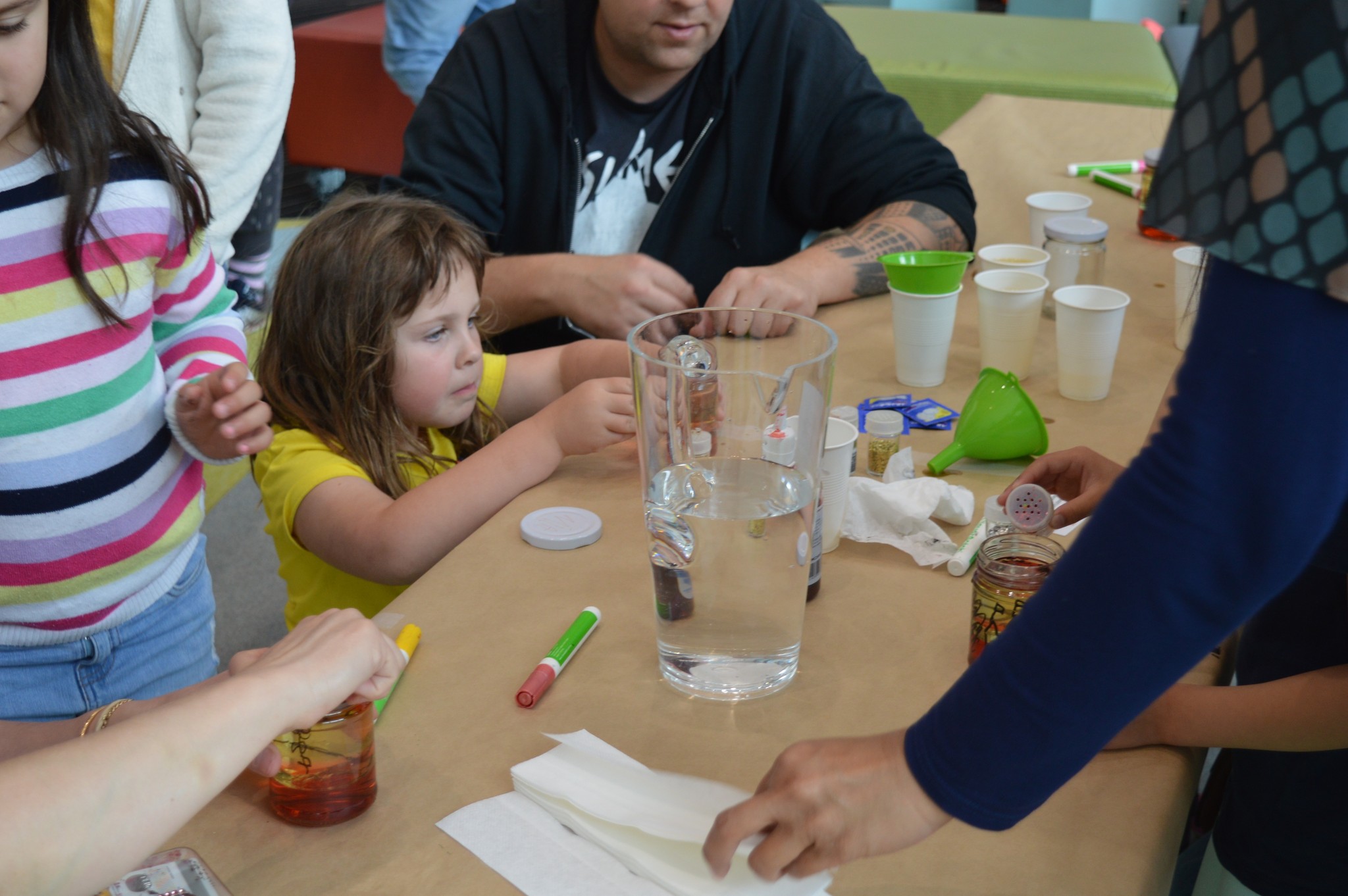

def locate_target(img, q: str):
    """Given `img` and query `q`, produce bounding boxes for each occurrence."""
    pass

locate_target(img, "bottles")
[1138,147,1186,242]
[764,426,824,602]
[1005,485,1053,537]
[1037,216,1109,322]
[828,405,860,473]
[865,409,904,477]
[967,532,1070,673]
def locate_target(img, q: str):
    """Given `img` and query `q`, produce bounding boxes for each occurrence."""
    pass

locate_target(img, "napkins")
[839,447,974,570]
[434,728,836,896]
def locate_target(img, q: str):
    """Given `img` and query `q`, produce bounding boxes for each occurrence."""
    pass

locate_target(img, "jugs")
[626,309,837,702]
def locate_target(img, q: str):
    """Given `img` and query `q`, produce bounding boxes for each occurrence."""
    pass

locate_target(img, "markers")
[948,517,997,576]
[369,622,422,726]
[1066,158,1145,179]
[1089,170,1142,199]
[514,606,601,710]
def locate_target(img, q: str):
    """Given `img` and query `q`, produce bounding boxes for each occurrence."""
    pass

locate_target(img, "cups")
[263,691,379,828]
[885,281,965,388]
[1053,285,1131,402]
[979,244,1051,282]
[767,415,860,554]
[1025,191,1092,251]
[1172,246,1209,352]
[974,269,1049,381]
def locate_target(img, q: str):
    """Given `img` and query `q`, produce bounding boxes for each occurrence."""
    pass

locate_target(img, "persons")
[401,0,980,394]
[701,1,1348,896]
[0,0,273,724]
[995,442,1348,896]
[69,1,307,277]
[239,189,683,655]
[376,0,516,115]
[0,601,406,896]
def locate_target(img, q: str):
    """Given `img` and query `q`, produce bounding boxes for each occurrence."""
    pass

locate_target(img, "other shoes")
[228,273,269,324]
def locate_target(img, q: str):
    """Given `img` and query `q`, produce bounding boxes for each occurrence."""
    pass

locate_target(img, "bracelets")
[82,695,134,738]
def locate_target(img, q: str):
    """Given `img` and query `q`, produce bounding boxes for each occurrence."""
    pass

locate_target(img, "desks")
[827,8,1180,137]
[90,95,1239,896]
[286,4,424,176]
